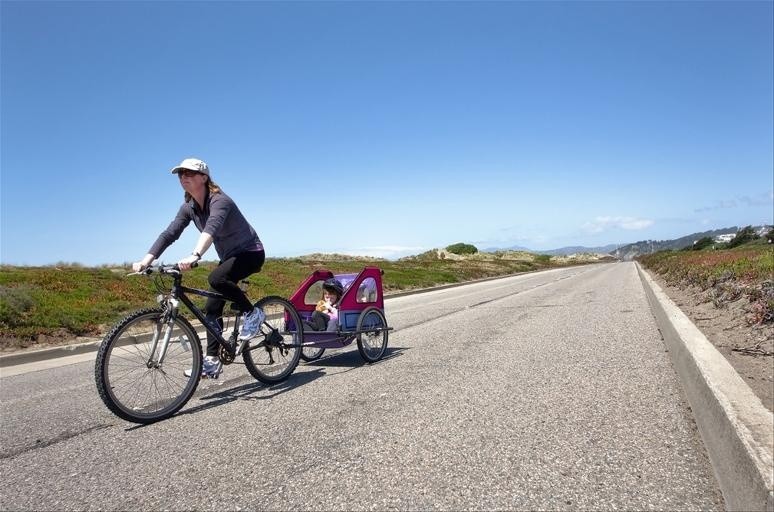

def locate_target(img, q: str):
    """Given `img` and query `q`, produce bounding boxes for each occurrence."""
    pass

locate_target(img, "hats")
[171,158,209,177]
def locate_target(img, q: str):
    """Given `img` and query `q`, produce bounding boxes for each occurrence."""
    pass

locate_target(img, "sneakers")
[184,356,223,377]
[239,307,266,341]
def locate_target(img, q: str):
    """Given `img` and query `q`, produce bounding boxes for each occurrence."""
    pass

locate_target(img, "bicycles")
[94,262,304,424]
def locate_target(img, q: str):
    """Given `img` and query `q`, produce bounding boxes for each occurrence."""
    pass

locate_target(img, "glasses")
[178,169,199,177]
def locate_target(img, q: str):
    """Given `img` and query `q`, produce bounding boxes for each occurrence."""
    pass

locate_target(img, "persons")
[131,158,266,378]
[301,277,368,332]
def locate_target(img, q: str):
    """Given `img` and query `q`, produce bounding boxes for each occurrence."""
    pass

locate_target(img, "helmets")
[323,278,343,294]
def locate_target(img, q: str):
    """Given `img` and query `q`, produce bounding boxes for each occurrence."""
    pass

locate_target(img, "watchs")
[190,252,201,261]
